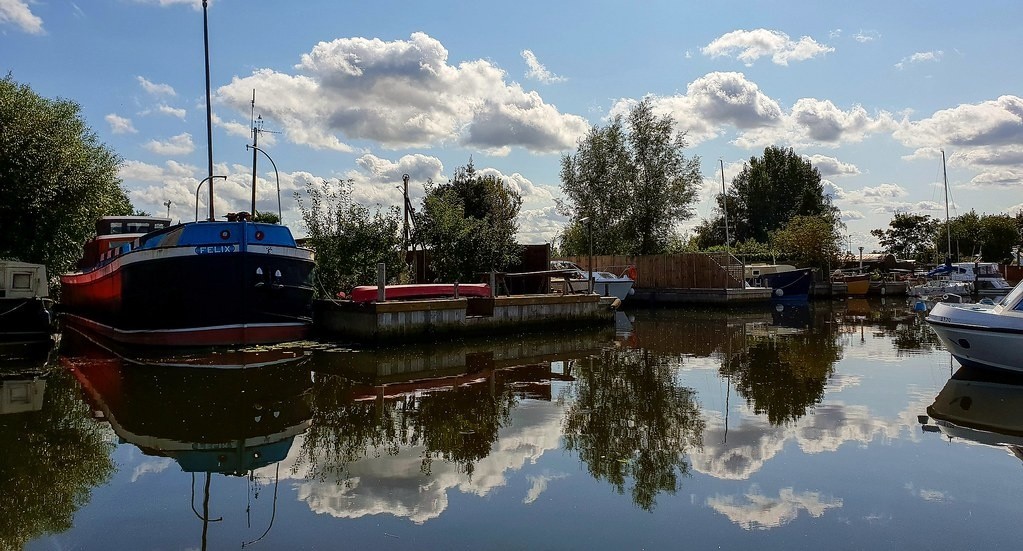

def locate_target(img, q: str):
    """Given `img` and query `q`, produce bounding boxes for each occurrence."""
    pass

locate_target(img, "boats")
[49,310,319,550]
[812,246,912,299]
[918,363,1023,460]
[58,143,317,344]
[923,278,1023,375]
[549,261,638,307]
[719,250,816,305]
[352,284,491,301]
[0,259,54,333]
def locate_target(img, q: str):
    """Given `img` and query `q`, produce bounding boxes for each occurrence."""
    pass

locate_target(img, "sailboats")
[906,148,1014,300]
